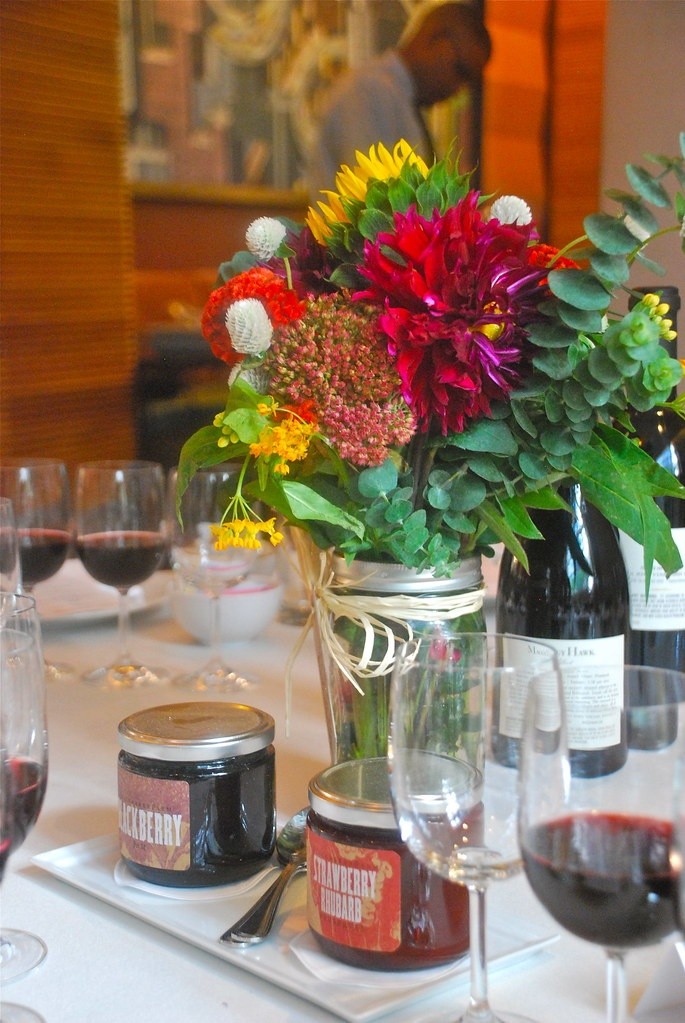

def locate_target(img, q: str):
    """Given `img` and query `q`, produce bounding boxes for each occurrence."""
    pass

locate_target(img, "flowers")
[171,143,685,578]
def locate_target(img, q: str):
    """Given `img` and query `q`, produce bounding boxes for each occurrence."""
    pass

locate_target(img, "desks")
[0,559,685,1023]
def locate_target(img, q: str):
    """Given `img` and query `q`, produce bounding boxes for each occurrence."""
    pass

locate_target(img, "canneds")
[116,701,277,888]
[305,747,484,972]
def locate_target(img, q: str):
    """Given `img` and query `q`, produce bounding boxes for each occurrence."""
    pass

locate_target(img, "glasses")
[449,37,484,87]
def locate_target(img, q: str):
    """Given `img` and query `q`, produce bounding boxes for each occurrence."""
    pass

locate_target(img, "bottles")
[613,284,684,751]
[487,465,628,779]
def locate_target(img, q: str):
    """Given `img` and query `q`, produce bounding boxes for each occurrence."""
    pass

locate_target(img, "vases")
[313,553,486,771]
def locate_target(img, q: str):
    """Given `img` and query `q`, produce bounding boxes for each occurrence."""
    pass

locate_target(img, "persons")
[310,4,492,203]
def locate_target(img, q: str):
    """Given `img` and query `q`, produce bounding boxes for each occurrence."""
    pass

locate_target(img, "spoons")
[217,805,308,949]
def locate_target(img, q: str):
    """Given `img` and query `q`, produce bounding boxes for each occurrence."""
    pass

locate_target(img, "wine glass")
[387,630,572,1023]
[73,459,169,691]
[517,663,685,1022]
[0,457,67,1022]
[168,463,261,693]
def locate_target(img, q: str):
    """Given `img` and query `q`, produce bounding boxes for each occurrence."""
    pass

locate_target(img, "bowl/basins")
[169,573,283,644]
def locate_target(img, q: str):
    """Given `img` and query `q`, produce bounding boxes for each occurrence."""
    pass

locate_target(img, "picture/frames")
[125,0,466,210]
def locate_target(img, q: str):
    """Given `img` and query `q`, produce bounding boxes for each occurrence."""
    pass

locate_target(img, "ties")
[412,97,437,169]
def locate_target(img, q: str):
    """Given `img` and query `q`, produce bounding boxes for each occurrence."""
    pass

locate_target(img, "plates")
[7,558,175,623]
[30,814,561,1022]
